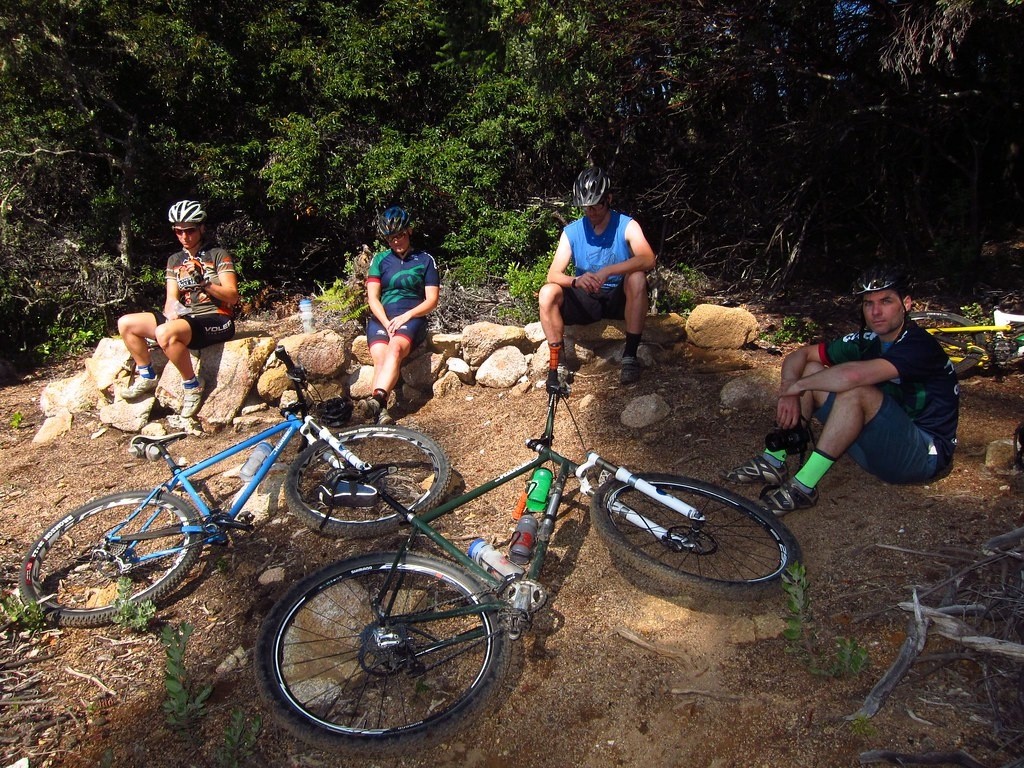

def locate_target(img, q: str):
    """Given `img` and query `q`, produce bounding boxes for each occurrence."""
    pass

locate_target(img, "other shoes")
[374,408,394,426]
[546,369,574,392]
[357,399,380,419]
[620,357,640,384]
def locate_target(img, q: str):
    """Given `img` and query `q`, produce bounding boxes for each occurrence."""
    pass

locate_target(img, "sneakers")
[756,479,819,518]
[180,383,205,418]
[120,375,159,398]
[718,455,789,489]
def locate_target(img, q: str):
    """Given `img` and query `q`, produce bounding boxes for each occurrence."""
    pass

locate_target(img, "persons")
[539,169,656,391]
[118,200,238,417]
[356,208,440,426]
[719,263,959,510]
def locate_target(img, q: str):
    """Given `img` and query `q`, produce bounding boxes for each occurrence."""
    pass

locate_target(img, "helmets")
[852,255,908,296]
[168,200,207,224]
[376,206,410,236]
[573,166,612,206]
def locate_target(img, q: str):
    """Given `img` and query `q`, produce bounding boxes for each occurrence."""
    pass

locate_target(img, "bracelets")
[572,279,576,288]
[200,278,209,291]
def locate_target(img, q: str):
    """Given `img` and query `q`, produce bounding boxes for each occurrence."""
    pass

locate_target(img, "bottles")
[509,514,539,565]
[239,440,274,483]
[468,538,525,582]
[525,467,553,512]
[299,300,316,334]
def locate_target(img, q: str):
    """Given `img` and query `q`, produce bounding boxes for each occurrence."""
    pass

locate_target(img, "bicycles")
[240,371,803,759]
[906,305,1024,378]
[13,345,452,627]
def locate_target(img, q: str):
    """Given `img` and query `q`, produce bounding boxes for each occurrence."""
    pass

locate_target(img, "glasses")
[172,224,202,235]
[581,198,607,212]
[384,230,408,242]
